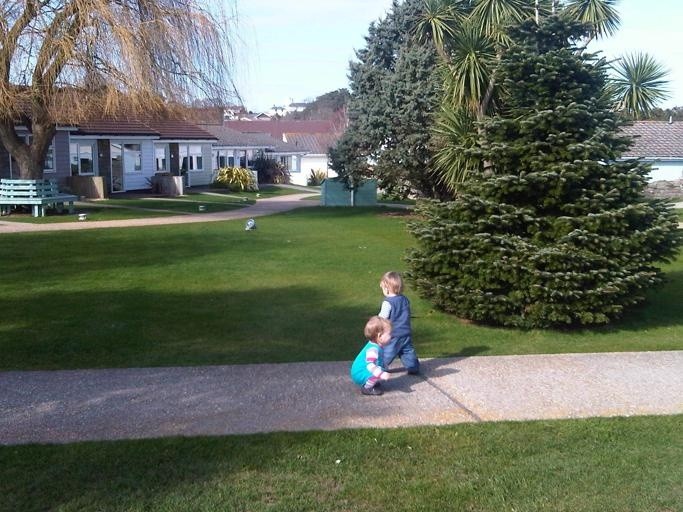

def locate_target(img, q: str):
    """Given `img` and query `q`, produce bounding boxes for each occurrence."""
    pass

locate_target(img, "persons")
[349,314,393,396]
[377,270,419,375]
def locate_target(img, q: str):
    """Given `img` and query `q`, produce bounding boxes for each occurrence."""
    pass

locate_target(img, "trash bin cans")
[319,177,351,206]
[353,178,377,206]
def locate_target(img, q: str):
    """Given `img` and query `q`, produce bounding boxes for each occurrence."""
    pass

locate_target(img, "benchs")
[0,177,79,218]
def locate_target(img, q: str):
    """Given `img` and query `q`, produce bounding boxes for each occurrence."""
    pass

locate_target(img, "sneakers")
[408,369,419,375]
[360,382,383,395]
